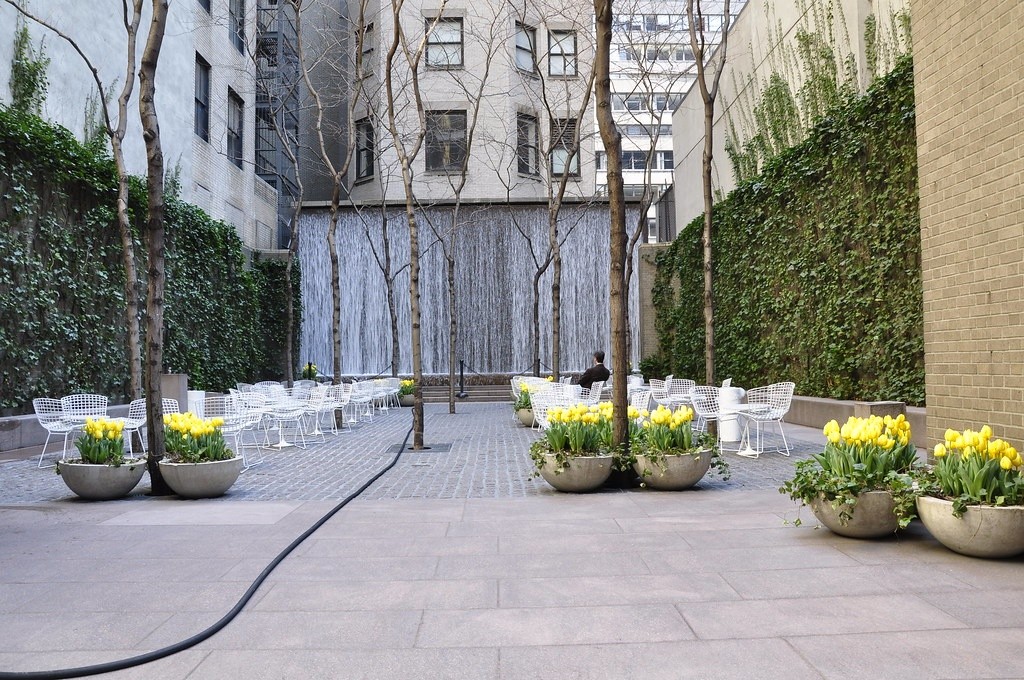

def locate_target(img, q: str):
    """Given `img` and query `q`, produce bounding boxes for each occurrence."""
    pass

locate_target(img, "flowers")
[401,378,414,395]
[74,417,125,465]
[933,424,1024,503]
[545,376,554,381]
[302,364,317,378]
[546,400,693,453]
[518,384,531,409]
[812,413,920,478]
[162,412,227,462]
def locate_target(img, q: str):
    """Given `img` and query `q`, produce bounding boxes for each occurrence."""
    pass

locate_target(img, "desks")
[726,403,772,456]
[670,393,704,409]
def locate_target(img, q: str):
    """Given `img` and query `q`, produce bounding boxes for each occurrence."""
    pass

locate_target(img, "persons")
[577,350,610,399]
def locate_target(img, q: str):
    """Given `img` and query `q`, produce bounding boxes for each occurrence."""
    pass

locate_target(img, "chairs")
[32,377,402,475]
[510,373,796,459]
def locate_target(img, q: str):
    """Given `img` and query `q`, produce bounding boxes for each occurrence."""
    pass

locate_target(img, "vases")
[912,479,1024,558]
[630,447,714,490]
[804,490,914,538]
[159,453,244,498]
[58,457,146,500]
[516,408,540,427]
[398,395,415,407]
[535,452,614,490]
